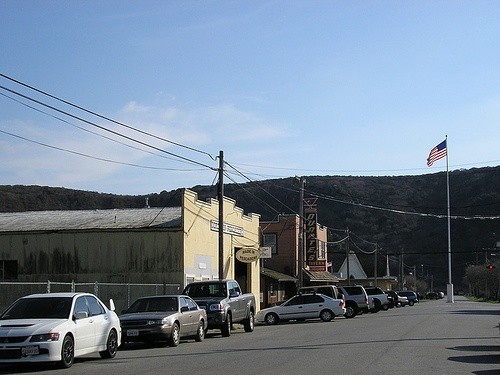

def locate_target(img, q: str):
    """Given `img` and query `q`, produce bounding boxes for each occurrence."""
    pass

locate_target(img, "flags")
[427,139,447,167]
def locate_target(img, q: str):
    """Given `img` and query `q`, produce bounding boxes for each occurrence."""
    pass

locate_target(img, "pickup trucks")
[180,279,257,336]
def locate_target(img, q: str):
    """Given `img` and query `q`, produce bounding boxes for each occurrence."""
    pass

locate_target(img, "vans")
[296,284,418,318]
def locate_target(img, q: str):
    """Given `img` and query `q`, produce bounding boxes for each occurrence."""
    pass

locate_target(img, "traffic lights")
[489,265,493,269]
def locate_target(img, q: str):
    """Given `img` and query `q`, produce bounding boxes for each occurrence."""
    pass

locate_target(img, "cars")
[257,294,346,326]
[425,291,444,300]
[118,295,209,347]
[0,291,122,368]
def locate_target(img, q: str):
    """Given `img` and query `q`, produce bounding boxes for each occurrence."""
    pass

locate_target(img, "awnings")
[260,267,297,281]
[303,268,339,283]
